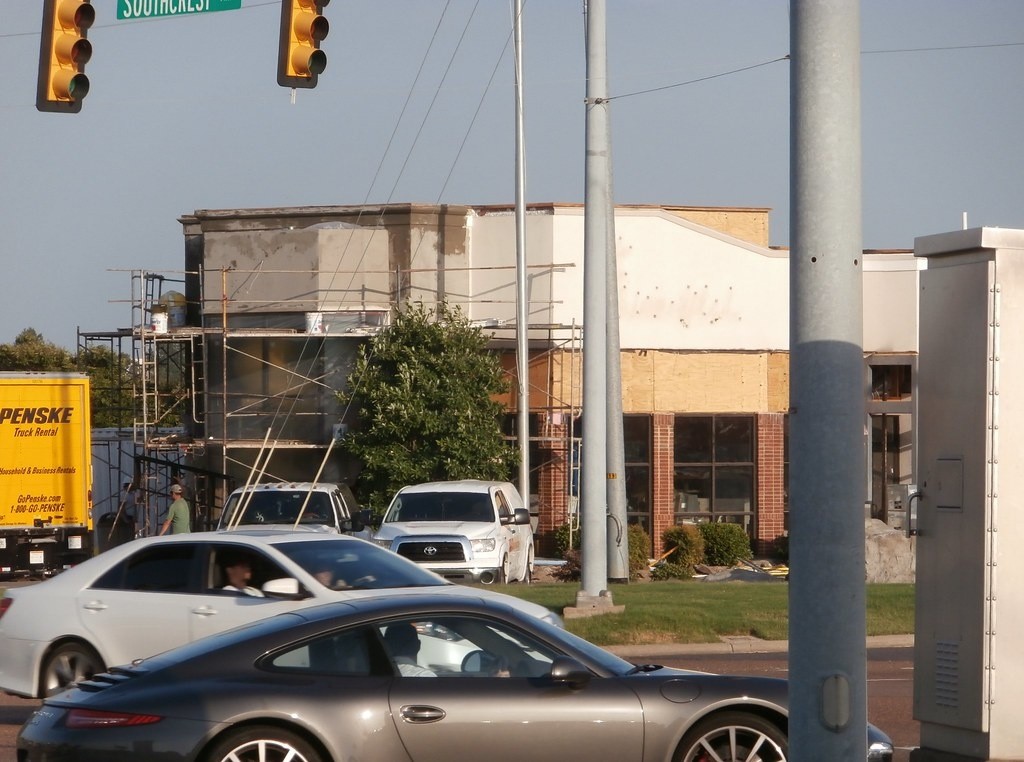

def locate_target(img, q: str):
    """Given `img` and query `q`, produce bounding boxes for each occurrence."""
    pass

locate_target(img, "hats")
[123,478,132,484]
[168,484,182,495]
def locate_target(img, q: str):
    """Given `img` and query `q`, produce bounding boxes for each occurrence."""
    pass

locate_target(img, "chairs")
[333,632,374,678]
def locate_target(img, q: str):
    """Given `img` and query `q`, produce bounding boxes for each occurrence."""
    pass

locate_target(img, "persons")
[385,623,512,677]
[221,551,349,599]
[158,485,190,535]
[119,477,138,541]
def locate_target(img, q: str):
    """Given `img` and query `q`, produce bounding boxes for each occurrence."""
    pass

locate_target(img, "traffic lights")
[276,0,331,89]
[36,0,95,113]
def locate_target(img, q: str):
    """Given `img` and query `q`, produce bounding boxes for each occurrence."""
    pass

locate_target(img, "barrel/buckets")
[332,423,348,441]
[150,302,168,333]
[169,306,186,328]
[304,312,323,333]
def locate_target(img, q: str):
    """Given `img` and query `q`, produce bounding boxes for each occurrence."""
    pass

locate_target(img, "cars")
[15,590,895,762]
[1,530,567,701]
[216,481,372,537]
[371,478,535,584]
